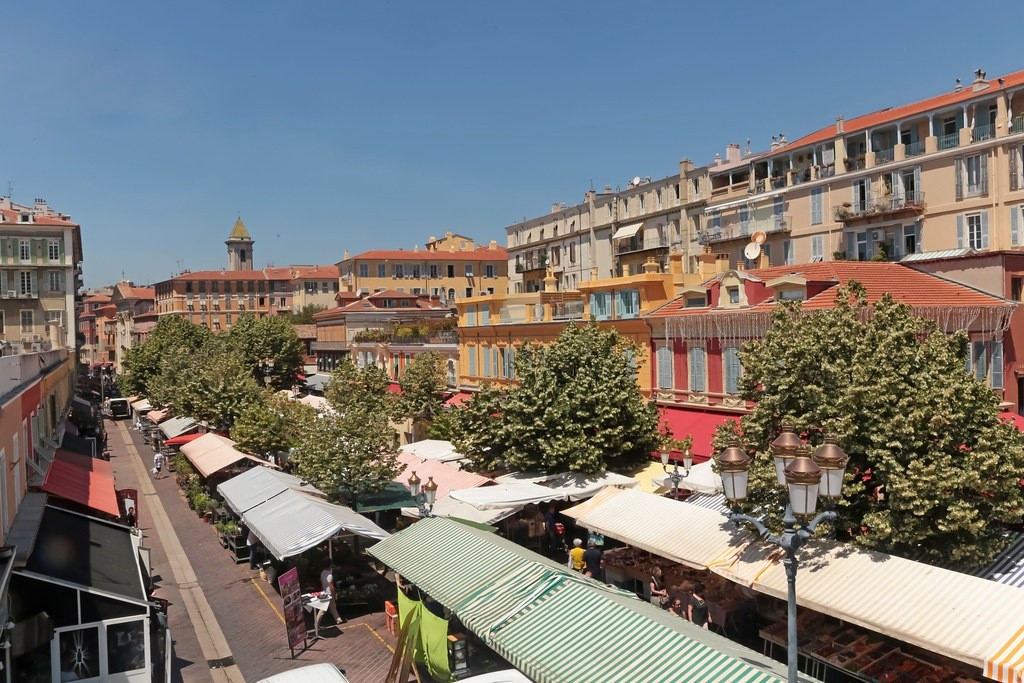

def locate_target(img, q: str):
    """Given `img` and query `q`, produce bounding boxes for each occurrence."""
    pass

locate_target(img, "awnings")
[44,447,120,517]
[133,389,1024,683]
[74,395,91,407]
[611,222,644,241]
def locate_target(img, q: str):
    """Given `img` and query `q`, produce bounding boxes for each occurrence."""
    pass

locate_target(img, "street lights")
[714,417,849,681]
[100,366,106,405]
[408,470,437,517]
[659,441,694,502]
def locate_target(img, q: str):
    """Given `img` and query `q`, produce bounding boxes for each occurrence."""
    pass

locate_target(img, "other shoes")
[250,565,260,570]
[340,619,347,625]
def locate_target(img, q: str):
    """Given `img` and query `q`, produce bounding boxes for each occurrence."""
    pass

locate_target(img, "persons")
[103,395,109,415]
[107,409,118,426]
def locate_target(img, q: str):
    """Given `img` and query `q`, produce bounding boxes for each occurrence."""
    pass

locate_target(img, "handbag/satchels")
[150,467,159,474]
[660,592,670,605]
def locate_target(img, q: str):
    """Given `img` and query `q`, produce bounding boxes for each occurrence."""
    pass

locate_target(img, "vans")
[109,398,131,418]
